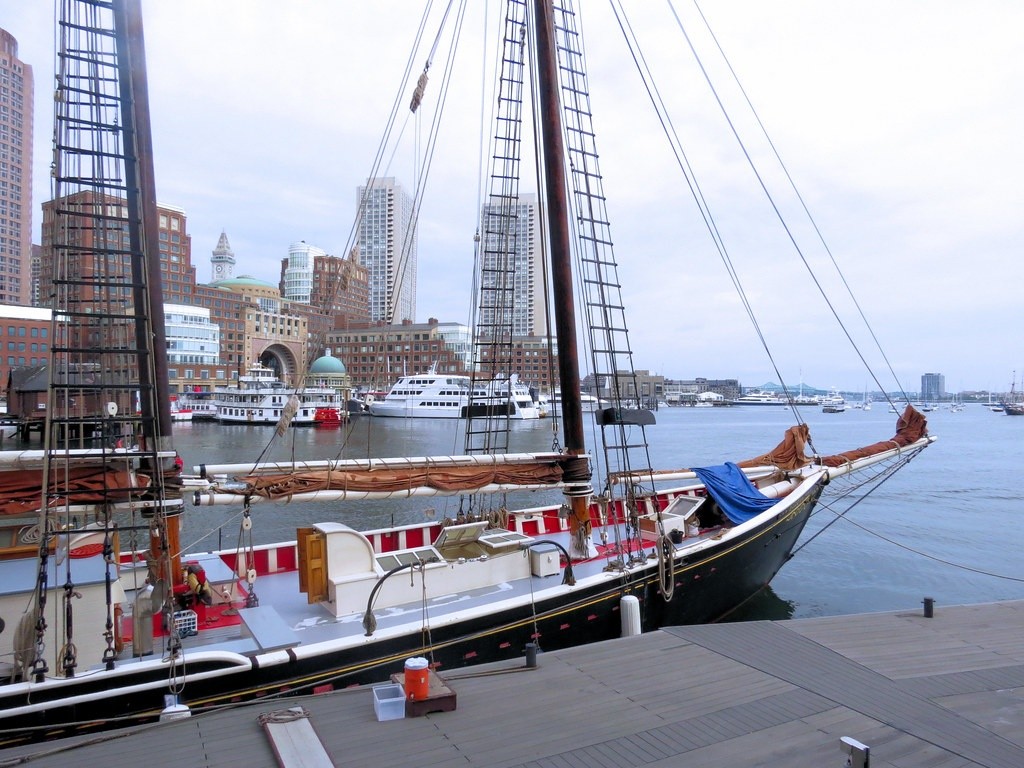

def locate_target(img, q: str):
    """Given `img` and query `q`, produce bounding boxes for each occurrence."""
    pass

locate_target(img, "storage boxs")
[636,493,706,542]
[372,683,407,721]
[531,544,561,578]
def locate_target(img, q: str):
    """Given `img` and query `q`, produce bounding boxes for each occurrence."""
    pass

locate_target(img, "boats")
[365,357,611,422]
[166,360,352,426]
[711,364,1024,417]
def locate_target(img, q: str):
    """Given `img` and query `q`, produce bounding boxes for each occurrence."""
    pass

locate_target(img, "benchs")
[90,638,261,675]
[237,604,302,650]
[199,558,239,605]
[118,553,220,590]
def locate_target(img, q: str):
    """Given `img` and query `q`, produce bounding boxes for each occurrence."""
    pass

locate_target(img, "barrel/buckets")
[404,657,428,700]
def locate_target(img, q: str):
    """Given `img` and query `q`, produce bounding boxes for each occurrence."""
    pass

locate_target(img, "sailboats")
[0,0,940,743]
